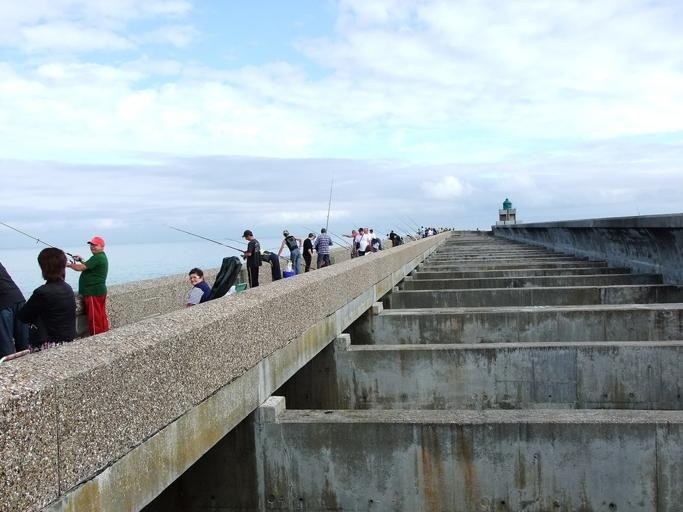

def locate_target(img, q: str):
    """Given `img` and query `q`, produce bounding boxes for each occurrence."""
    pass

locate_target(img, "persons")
[0,263,33,359]
[343,226,454,259]
[187,268,210,307]
[242,230,262,288]
[66,237,108,336]
[16,248,77,348]
[278,230,302,274]
[303,233,316,272]
[314,228,333,269]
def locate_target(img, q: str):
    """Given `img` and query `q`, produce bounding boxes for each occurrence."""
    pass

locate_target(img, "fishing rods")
[372,214,423,236]
[169,226,247,253]
[326,176,335,231]
[326,228,352,249]
[308,229,349,251]
[227,239,316,272]
[1,222,87,263]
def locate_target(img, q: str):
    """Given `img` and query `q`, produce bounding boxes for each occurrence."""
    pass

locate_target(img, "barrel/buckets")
[282,269,295,277]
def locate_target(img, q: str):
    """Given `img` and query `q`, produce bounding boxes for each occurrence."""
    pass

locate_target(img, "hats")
[283,229,289,235]
[242,230,253,237]
[87,237,105,247]
